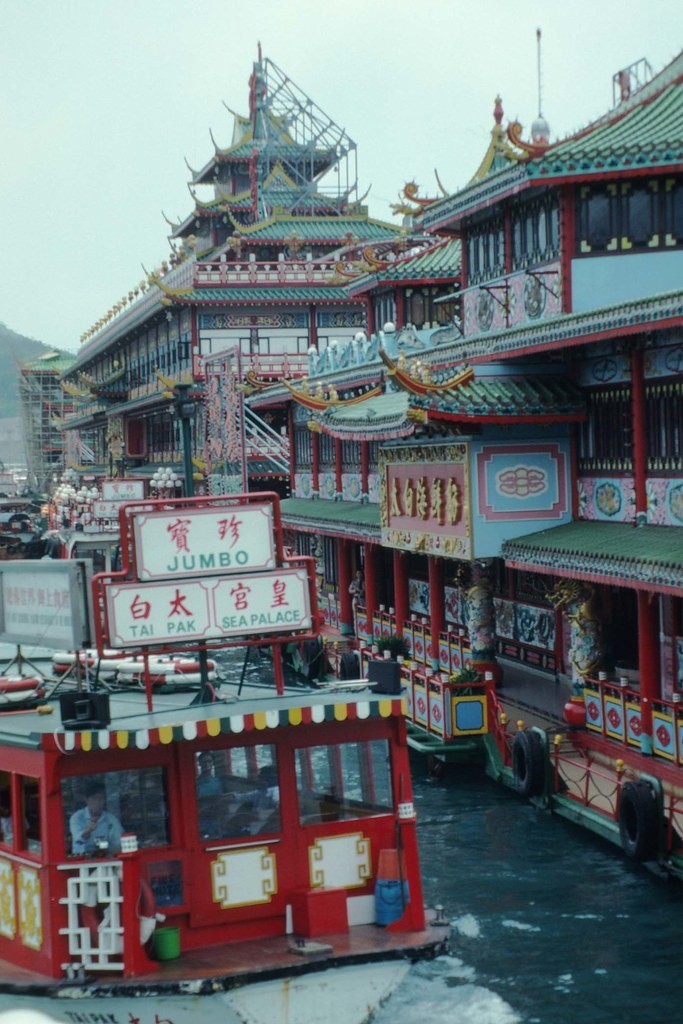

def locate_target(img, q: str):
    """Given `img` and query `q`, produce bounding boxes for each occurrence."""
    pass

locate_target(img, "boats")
[52,646,218,695]
[0,480,178,640]
[0,652,453,1024]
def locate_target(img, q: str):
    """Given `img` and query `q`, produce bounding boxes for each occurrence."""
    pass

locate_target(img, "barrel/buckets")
[151,925,181,960]
[376,849,407,882]
[374,880,410,926]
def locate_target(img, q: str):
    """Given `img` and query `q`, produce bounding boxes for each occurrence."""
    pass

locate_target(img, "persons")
[349,570,366,640]
[68,789,129,856]
[196,753,223,800]
[220,767,281,824]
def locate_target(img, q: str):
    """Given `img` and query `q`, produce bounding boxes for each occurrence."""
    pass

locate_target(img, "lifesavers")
[0,674,44,704]
[52,647,217,686]
